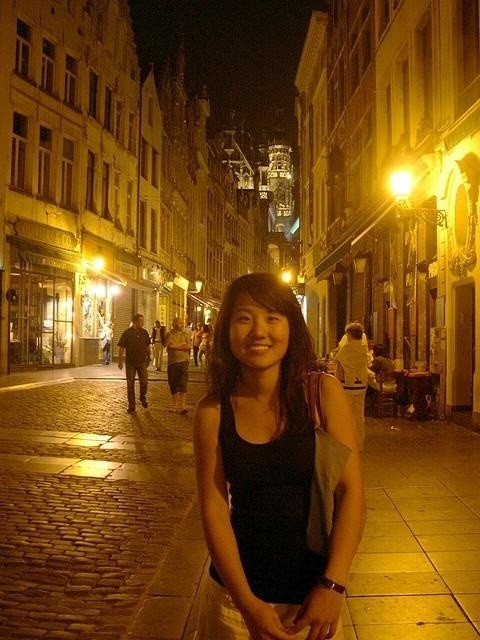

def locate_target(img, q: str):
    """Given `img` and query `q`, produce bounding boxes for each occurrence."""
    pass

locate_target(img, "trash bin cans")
[404,372,440,421]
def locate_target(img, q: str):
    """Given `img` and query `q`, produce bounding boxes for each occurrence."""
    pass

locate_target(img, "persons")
[338,319,369,356]
[162,316,193,415]
[191,271,368,639]
[118,313,151,413]
[365,341,399,396]
[97,316,215,370]
[334,322,370,454]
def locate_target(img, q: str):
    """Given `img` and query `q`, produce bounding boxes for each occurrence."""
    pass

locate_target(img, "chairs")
[366,368,397,418]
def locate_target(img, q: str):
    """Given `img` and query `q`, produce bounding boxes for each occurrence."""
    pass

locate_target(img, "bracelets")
[319,574,347,595]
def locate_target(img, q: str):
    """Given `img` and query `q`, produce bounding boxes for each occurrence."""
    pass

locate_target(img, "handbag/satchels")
[303,427,353,556]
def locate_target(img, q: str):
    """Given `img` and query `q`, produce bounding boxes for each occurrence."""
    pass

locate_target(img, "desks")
[398,370,437,423]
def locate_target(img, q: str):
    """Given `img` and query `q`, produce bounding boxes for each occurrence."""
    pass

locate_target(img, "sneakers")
[154,368,161,372]
[180,408,188,416]
[168,408,178,414]
[139,396,149,409]
[127,407,136,413]
